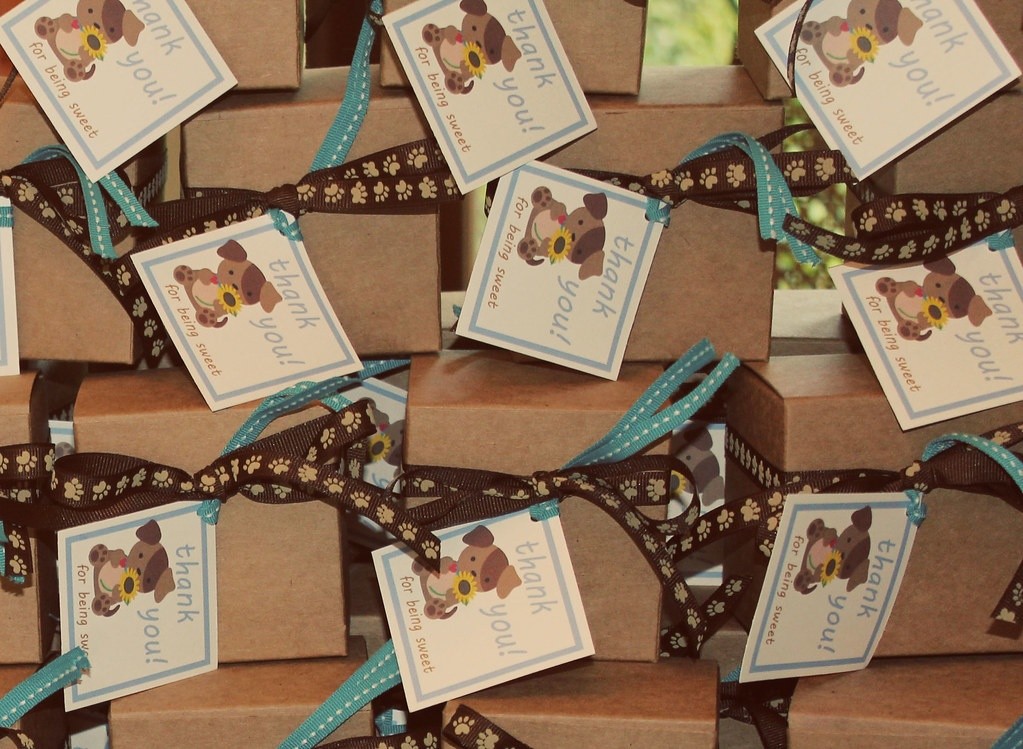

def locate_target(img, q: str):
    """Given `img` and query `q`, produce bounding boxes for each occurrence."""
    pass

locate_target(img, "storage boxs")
[0,1,1023,749]
